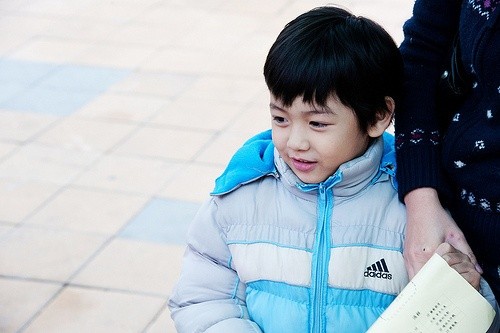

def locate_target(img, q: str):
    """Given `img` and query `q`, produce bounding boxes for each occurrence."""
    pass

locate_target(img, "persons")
[394,0,499,310]
[169,6,499,333]
